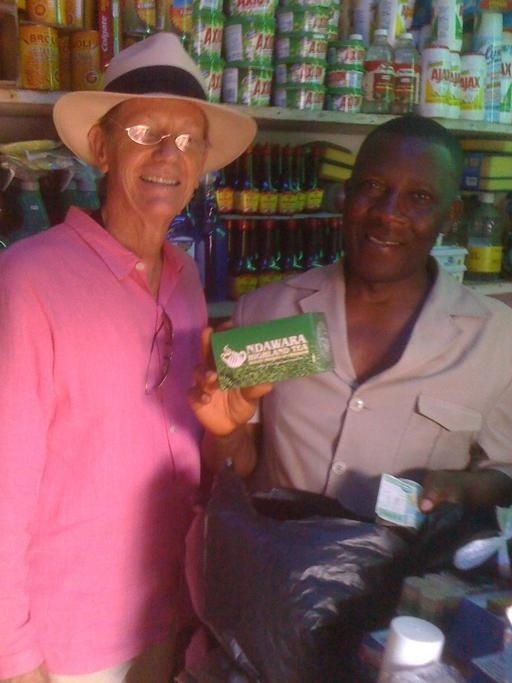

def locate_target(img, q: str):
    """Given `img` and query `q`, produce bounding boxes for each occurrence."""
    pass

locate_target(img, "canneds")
[18,0,102,92]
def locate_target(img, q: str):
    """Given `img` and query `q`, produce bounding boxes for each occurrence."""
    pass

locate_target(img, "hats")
[52,30,257,173]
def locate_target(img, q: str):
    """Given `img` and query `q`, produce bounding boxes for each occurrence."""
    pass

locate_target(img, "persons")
[188,115,510,511]
[2,33,256,682]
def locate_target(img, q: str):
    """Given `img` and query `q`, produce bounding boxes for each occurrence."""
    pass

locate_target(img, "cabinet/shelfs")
[1,86,511,320]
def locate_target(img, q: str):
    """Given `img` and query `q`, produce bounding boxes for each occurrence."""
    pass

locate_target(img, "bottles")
[214,144,347,300]
[10,173,96,245]
[462,192,505,279]
[362,28,422,116]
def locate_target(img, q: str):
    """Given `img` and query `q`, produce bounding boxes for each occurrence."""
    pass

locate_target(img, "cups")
[373,614,446,679]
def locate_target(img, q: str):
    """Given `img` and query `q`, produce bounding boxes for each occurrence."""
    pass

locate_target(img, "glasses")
[107,114,214,158]
